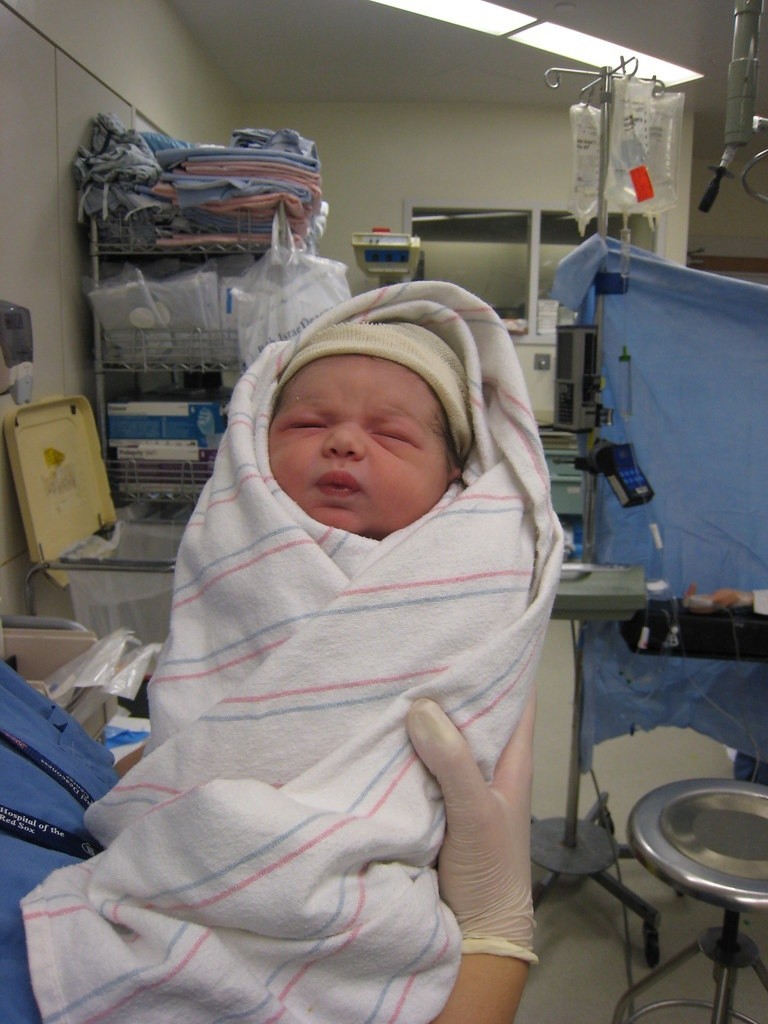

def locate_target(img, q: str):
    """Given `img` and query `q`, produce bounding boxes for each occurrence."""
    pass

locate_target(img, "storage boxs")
[0,627,118,740]
[107,389,229,461]
[220,277,253,363]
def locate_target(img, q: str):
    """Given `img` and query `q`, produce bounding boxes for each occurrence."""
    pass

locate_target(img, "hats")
[273,322,473,465]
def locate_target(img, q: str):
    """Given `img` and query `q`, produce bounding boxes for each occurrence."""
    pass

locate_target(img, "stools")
[614,781,768,1024]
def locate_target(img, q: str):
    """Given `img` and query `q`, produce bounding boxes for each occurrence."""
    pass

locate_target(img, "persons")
[0,658,540,1023]
[20,280,565,1024]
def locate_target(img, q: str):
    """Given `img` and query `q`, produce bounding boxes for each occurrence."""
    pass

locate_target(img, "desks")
[530,569,690,968]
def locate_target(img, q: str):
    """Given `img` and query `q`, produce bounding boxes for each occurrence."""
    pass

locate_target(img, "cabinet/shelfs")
[91,206,306,502]
